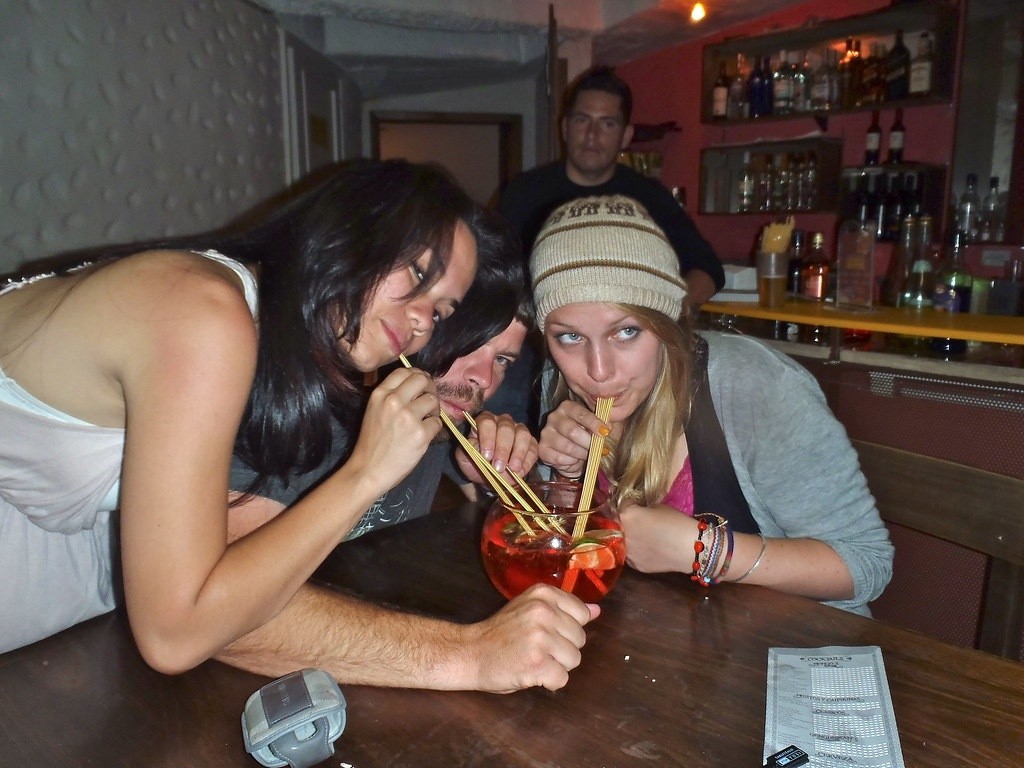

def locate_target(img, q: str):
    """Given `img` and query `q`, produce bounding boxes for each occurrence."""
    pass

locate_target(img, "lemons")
[503,515,623,570]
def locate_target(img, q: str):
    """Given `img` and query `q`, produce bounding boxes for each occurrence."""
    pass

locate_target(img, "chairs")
[845,436,1024,664]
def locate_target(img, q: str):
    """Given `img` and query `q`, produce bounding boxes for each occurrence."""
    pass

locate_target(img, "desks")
[0,493,1024,768]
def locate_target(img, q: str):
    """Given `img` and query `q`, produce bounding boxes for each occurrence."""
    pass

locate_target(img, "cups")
[482,482,627,605]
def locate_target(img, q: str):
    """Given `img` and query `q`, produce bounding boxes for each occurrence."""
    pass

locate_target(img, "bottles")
[864,110,881,167]
[700,143,818,215]
[756,224,876,350]
[710,27,942,122]
[887,109,906,165]
[950,173,1010,242]
[841,167,927,242]
[880,215,1024,354]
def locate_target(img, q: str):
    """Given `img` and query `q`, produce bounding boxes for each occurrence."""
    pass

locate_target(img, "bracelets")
[732,533,767,583]
[691,512,734,586]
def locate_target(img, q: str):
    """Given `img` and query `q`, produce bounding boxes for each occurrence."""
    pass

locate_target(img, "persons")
[211,202,601,693]
[0,159,516,675]
[528,195,895,618]
[497,67,725,314]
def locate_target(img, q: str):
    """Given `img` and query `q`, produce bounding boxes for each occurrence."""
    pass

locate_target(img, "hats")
[528,192,688,336]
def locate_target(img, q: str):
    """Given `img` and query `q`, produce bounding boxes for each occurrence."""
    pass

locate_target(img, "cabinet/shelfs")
[613,0,1024,318]
